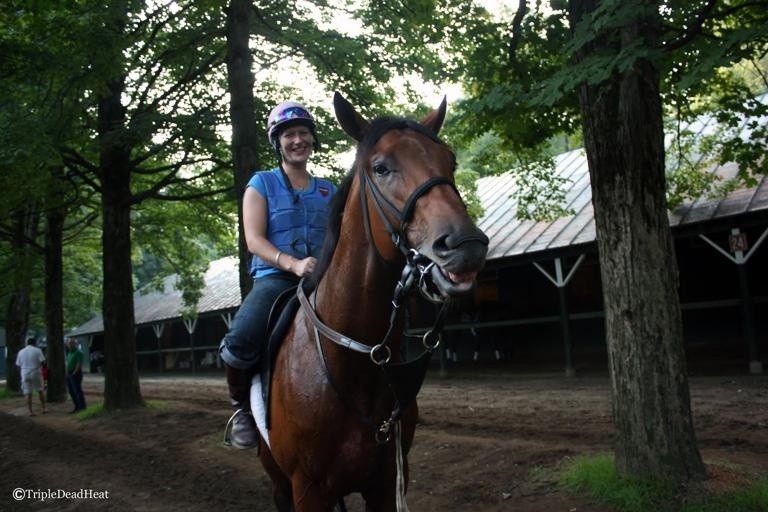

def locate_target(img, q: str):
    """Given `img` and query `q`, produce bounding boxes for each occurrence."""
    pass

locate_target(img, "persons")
[218,99,345,452]
[15,337,51,418]
[39,346,48,393]
[63,336,87,414]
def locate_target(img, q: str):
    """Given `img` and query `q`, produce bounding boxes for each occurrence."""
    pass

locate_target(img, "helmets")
[267,101,314,135]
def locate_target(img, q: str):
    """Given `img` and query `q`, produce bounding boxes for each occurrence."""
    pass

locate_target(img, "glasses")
[268,106,314,129]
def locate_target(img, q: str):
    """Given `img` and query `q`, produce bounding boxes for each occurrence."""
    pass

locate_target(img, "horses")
[249,91,490,512]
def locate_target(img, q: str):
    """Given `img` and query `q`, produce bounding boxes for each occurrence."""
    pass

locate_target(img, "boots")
[223,361,259,450]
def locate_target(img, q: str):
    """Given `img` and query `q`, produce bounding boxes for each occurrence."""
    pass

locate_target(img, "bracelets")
[274,250,284,270]
